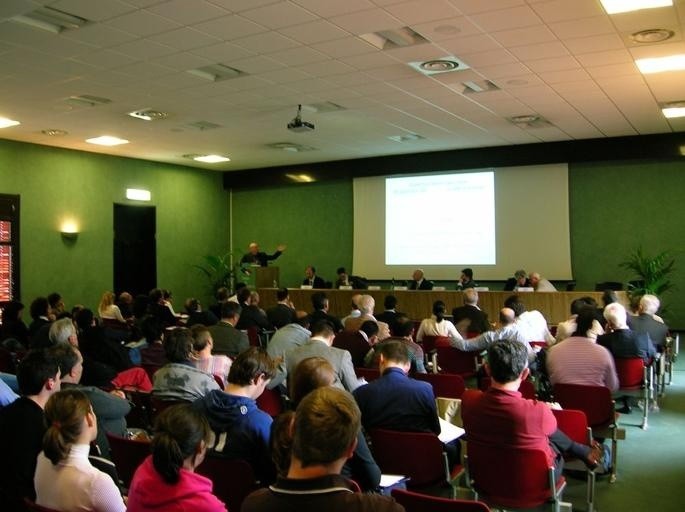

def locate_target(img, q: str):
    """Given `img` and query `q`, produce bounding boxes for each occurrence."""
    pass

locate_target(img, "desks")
[257,287,641,331]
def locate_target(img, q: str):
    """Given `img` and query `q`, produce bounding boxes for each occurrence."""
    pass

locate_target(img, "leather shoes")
[586,441,601,470]
[625,406,633,413]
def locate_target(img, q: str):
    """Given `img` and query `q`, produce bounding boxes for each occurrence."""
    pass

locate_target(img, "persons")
[503,270,529,291]
[240,242,287,276]
[456,267,479,292]
[0,288,464,512]
[303,265,325,289]
[334,267,368,289]
[410,270,435,290]
[447,288,666,511]
[529,271,557,292]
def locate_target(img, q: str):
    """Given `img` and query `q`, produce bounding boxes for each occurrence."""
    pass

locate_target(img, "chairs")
[595,282,623,290]
[504,278,529,290]
[349,275,368,289]
[628,280,645,290]
[566,284,576,291]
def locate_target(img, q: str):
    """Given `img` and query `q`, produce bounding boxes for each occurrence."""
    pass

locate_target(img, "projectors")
[287,121,314,134]
